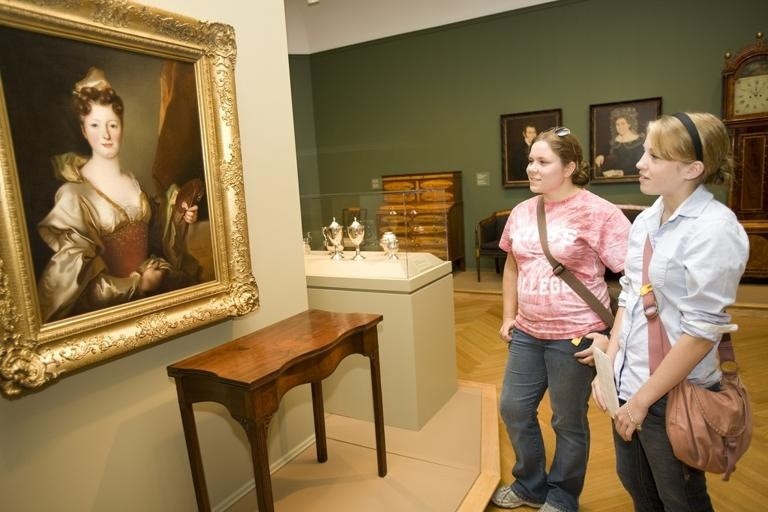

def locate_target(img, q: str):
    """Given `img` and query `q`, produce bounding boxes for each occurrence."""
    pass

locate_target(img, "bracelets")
[626,398,642,431]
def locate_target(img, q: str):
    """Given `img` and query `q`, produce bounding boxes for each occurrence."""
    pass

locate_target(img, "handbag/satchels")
[666,371,753,480]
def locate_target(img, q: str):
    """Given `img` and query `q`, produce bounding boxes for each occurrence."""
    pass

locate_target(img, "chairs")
[475,209,511,282]
[342,207,367,238]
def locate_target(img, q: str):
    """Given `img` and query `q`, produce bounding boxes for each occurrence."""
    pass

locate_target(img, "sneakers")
[493,487,560,512]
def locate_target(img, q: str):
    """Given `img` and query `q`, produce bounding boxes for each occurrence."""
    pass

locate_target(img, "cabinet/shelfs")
[729,122,768,283]
[376,170,466,274]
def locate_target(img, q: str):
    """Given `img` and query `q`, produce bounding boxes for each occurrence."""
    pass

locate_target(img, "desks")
[308,250,457,431]
[166,307,388,509]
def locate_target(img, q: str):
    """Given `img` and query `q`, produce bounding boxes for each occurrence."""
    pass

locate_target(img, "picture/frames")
[587,96,663,187]
[0,0,262,402]
[501,106,563,189]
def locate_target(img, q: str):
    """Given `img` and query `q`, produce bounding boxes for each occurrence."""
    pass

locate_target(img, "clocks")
[732,75,768,116]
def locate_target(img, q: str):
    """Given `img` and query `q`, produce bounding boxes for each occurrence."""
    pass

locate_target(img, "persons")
[38,67,204,321]
[491,127,634,512]
[516,124,537,181]
[595,106,645,177]
[591,109,751,512]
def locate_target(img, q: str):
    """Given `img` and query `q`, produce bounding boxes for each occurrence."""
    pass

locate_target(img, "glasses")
[544,127,571,137]
